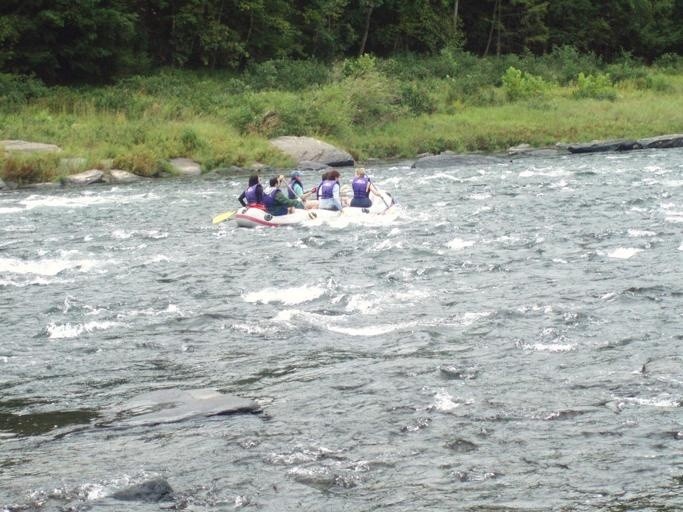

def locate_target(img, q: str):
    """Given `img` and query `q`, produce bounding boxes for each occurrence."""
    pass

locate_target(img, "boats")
[236,190,394,227]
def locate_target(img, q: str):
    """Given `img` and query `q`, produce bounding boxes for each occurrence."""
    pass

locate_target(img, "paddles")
[280,176,317,219]
[213,205,244,224]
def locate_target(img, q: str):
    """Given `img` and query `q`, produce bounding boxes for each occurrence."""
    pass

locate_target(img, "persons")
[317,170,341,210]
[316,172,340,200]
[261,175,302,215]
[238,175,265,207]
[287,170,320,210]
[350,167,381,207]
